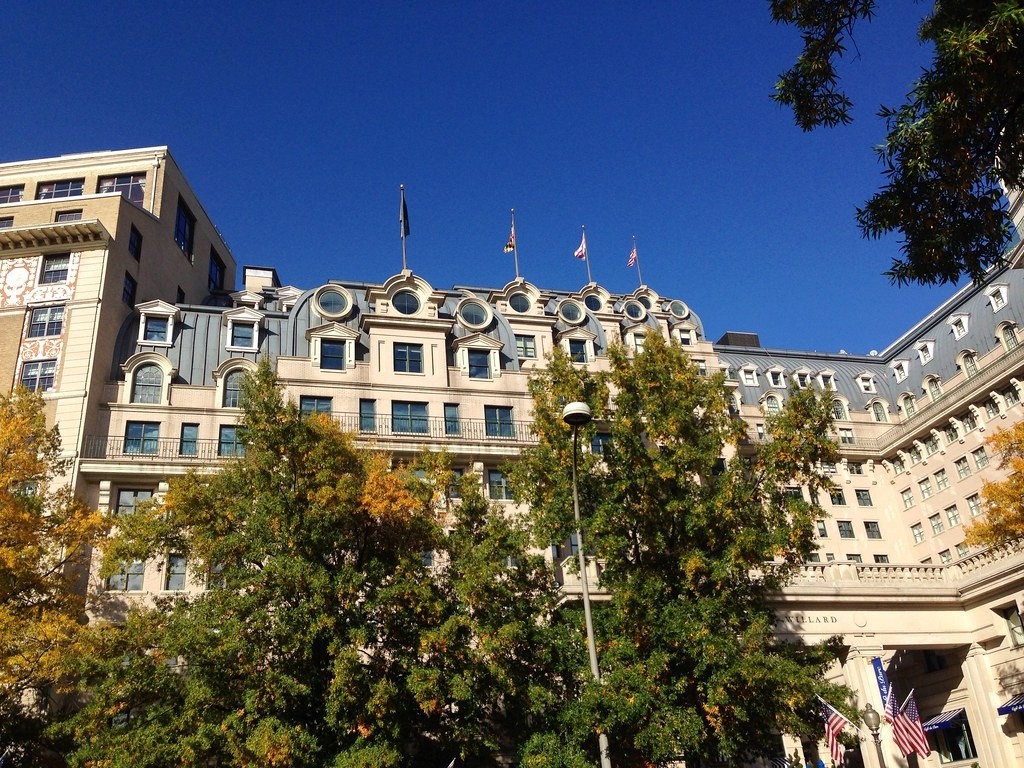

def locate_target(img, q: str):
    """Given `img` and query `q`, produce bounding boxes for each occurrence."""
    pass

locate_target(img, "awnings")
[997,694,1024,716]
[922,708,965,732]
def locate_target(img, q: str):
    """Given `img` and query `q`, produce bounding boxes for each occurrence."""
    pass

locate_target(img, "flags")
[885,681,932,760]
[626,248,637,268]
[400,183,410,240]
[819,699,848,763]
[573,233,587,262]
[503,216,514,253]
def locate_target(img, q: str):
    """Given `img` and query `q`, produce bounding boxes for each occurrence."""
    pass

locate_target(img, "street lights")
[561,400,614,768]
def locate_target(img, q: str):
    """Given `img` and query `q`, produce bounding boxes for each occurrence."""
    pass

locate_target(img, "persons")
[806,759,816,768]
